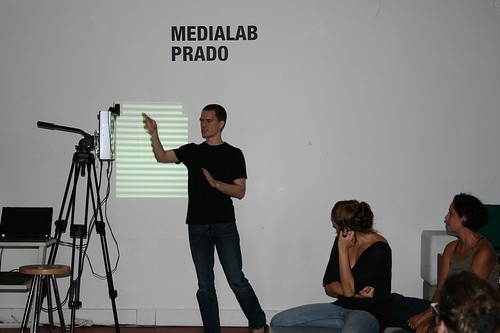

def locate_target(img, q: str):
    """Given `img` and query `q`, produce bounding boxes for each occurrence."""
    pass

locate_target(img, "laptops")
[0,206,54,242]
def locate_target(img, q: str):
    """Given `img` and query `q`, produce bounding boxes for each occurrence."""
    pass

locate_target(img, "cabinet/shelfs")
[0,239,58,333]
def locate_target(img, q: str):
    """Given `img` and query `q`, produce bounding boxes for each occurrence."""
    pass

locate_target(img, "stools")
[19,264,70,332]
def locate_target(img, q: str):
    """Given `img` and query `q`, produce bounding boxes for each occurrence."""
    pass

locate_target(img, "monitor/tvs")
[100,111,117,159]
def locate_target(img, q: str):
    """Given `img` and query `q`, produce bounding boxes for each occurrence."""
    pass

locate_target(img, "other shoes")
[249,325,269,332]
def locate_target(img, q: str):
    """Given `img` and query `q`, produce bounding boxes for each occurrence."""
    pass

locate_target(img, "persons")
[270,200,392,333]
[430,271,500,333]
[391,193,500,333]
[142,104,270,333]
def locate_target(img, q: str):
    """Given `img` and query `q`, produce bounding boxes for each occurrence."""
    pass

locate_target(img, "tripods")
[37,120,121,333]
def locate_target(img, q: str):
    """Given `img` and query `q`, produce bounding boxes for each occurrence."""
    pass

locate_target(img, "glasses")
[430,301,443,318]
